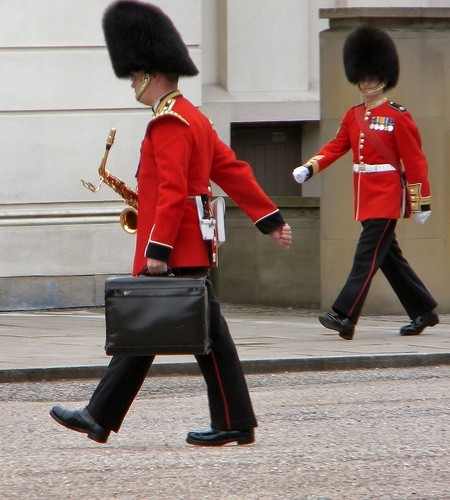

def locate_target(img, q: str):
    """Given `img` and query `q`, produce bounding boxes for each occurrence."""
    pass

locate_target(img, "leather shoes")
[49,406,111,444]
[319,312,355,339]
[400,310,439,336]
[186,428,256,447]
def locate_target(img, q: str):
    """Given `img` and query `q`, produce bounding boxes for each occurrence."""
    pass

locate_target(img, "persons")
[292,28,439,339]
[50,0,293,447]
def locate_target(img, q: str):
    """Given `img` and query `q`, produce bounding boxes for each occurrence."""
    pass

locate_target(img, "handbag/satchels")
[212,197,226,246]
[104,266,213,357]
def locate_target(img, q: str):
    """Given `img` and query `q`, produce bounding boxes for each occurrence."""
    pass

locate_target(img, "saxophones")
[97,127,139,234]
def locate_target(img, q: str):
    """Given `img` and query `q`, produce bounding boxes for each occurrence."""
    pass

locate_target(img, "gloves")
[292,166,309,185]
[416,210,431,223]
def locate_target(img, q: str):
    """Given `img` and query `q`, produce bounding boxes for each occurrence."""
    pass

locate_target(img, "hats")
[343,26,400,92]
[101,0,200,77]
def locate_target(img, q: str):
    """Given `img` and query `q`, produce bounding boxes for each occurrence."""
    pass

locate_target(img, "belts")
[353,163,397,173]
